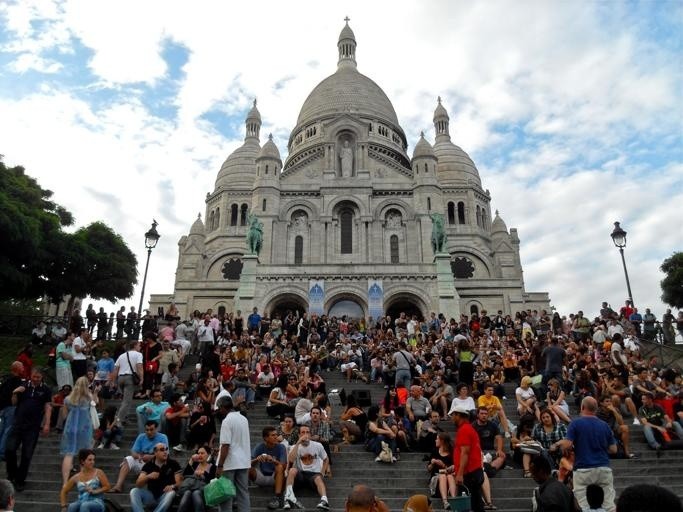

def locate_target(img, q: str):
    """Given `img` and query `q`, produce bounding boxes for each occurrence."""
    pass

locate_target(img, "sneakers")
[173,444,185,451]
[110,443,119,449]
[97,441,105,450]
[269,494,330,509]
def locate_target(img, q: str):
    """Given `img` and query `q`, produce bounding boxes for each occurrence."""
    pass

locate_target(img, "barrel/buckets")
[447,484,471,512]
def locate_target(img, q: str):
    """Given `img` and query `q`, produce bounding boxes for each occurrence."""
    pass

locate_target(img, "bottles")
[397,421,402,429]
[461,491,467,496]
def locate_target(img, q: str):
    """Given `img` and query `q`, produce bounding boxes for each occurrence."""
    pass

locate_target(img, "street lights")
[132,221,160,339]
[610,221,635,308]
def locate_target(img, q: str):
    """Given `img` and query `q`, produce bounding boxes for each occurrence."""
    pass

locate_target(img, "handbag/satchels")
[520,443,540,456]
[410,365,420,378]
[203,476,237,506]
[380,442,393,462]
[145,360,157,372]
[180,474,201,492]
[132,371,140,385]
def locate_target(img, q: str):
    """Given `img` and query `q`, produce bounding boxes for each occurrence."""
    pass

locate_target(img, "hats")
[449,406,470,417]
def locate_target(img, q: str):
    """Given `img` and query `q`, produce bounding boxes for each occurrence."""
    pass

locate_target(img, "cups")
[425,407,429,413]
[260,453,267,463]
[390,410,394,416]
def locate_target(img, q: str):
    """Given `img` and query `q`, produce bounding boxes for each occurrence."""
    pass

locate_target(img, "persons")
[427,210,447,253]
[2,302,682,510]
[340,140,353,177]
[246,216,261,255]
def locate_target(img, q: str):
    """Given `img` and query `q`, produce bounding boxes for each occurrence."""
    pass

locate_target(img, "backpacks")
[48,340,67,366]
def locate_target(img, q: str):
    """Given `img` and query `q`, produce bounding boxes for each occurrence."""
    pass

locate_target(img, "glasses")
[157,447,169,451]
